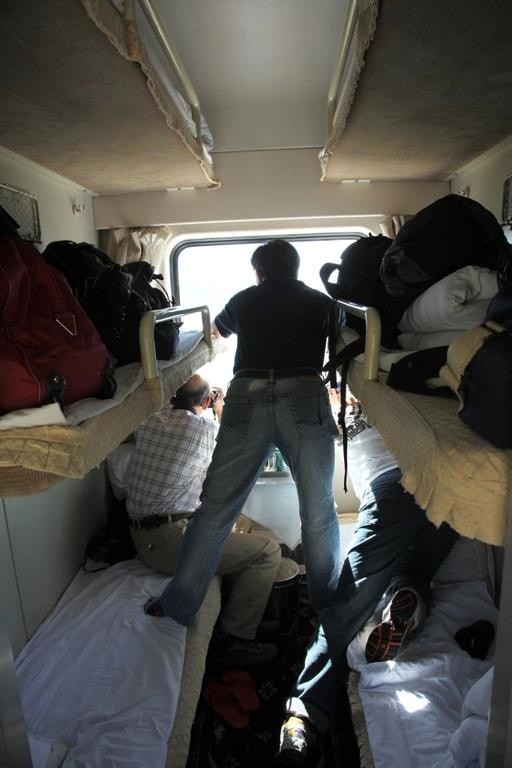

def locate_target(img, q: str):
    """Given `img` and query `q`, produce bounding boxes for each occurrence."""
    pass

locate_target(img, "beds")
[332,295,511,548]
[337,512,501,766]
[316,1,511,184]
[2,300,218,479]
[14,561,223,767]
[2,0,224,199]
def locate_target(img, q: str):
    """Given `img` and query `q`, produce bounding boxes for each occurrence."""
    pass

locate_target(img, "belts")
[126,512,196,531]
[232,368,318,379]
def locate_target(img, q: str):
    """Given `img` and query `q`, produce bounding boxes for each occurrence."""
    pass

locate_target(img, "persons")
[142,236,348,629]
[271,378,464,768]
[122,372,282,667]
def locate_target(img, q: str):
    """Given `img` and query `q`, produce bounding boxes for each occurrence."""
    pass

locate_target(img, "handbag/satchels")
[81,520,125,573]
[0,205,177,414]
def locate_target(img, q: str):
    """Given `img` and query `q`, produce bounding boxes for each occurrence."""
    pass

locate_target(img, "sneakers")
[365,588,426,663]
[208,632,277,666]
[284,696,331,737]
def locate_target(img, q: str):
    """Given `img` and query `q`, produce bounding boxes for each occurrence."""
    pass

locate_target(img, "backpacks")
[320,231,402,351]
[384,307,512,449]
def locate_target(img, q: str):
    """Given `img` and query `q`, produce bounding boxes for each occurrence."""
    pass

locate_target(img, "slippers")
[201,669,260,730]
